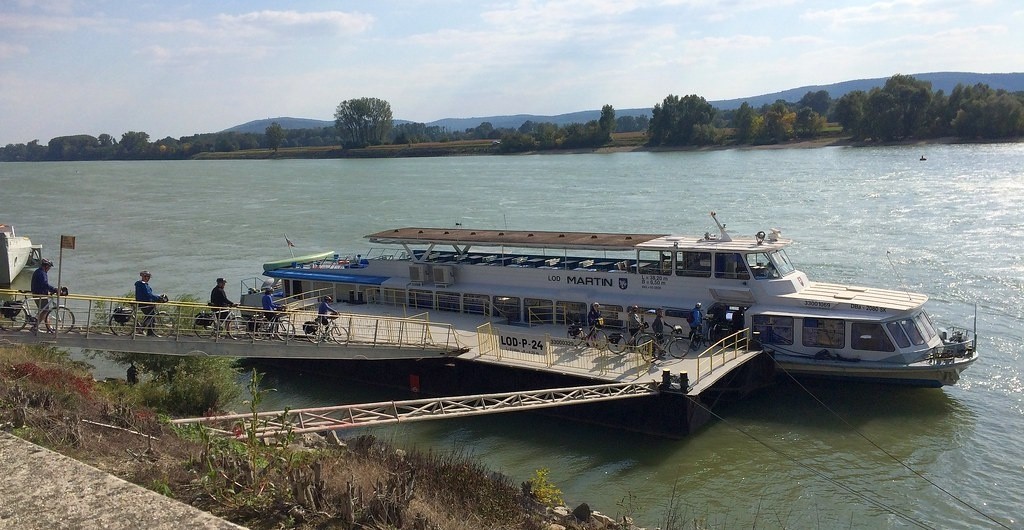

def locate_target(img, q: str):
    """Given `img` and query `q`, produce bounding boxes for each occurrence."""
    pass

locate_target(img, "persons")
[29,258,68,334]
[585,302,605,347]
[652,308,676,360]
[630,305,644,351]
[732,307,744,330]
[134,271,163,337]
[687,303,703,340]
[262,286,286,339]
[319,295,339,342]
[714,304,726,340]
[211,278,238,340]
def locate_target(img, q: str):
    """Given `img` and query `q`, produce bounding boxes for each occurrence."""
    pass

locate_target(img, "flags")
[286,238,295,248]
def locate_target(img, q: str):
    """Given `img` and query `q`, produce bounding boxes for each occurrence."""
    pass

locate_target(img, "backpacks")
[686,310,697,323]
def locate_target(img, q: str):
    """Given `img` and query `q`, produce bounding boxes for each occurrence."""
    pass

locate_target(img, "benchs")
[428,252,631,272]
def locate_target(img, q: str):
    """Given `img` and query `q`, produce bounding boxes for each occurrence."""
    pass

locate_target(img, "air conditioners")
[432,266,454,284]
[409,264,429,282]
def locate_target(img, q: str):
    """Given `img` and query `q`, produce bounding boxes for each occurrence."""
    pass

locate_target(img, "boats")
[0,225,42,288]
[238,210,984,389]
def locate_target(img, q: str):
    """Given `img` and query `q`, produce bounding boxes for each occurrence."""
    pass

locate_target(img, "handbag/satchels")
[114,307,133,323]
[248,318,258,333]
[689,330,697,341]
[3,300,22,318]
[568,323,582,336]
[195,313,214,326]
[303,321,316,334]
[609,333,622,345]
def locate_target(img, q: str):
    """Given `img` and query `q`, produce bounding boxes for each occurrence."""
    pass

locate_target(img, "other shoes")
[137,330,146,336]
[48,327,56,333]
[651,353,657,358]
[585,342,590,347]
[595,344,598,346]
[29,327,41,332]
[147,335,158,337]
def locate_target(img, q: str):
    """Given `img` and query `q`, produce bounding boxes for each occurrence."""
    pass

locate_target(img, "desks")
[414,253,425,258]
[436,254,651,275]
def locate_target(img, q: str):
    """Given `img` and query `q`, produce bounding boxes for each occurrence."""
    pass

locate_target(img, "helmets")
[631,304,638,311]
[139,270,151,276]
[695,303,701,309]
[216,278,227,283]
[42,258,54,266]
[593,303,600,307]
[266,287,274,291]
[324,295,332,301]
[655,308,664,315]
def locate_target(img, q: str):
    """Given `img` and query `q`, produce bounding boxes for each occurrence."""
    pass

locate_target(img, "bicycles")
[641,325,690,363]
[303,310,349,345]
[689,317,726,352]
[244,306,296,341]
[109,297,176,337]
[608,324,656,356]
[0,290,75,335]
[193,302,248,340]
[566,317,607,350]
